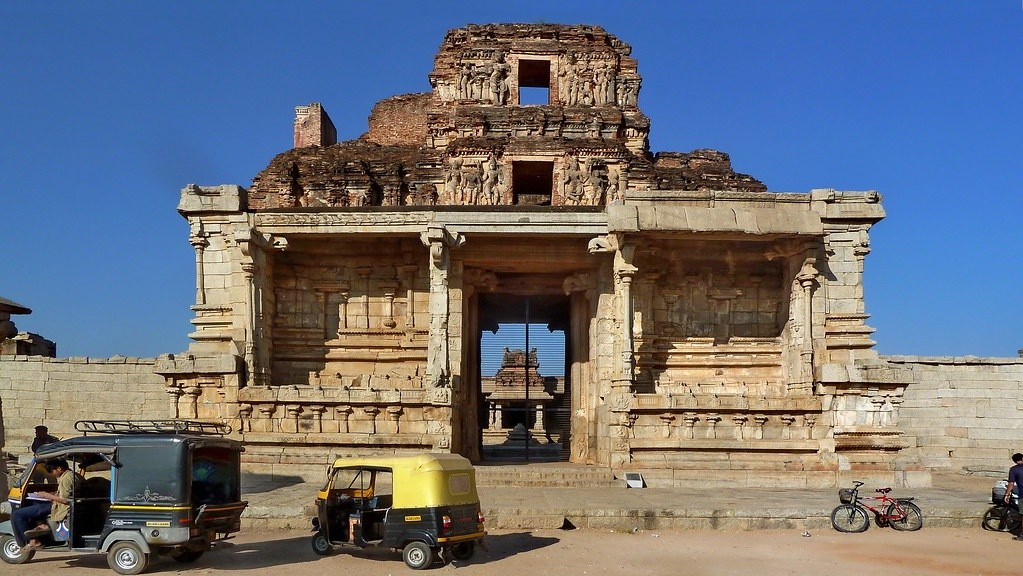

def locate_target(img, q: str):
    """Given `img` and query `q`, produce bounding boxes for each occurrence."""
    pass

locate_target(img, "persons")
[561,53,581,107]
[1003,453,1023,538]
[487,52,512,105]
[480,158,500,206]
[445,160,464,204]
[30,425,61,452]
[592,59,616,106]
[11,456,86,553]
[562,158,585,206]
[459,63,475,97]
[587,170,608,205]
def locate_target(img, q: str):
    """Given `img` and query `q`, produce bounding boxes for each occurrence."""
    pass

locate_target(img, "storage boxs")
[992,487,1006,501]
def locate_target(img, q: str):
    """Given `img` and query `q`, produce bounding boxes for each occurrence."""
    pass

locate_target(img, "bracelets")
[1005,494,1010,496]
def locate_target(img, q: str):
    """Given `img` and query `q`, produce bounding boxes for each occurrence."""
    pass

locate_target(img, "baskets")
[839,489,851,503]
[992,486,1008,503]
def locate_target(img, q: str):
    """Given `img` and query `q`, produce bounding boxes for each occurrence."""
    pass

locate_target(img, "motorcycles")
[311,454,488,570]
[0,420,249,575]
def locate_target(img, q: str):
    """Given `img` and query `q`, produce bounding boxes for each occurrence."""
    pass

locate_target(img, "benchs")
[49,477,111,526]
[358,494,393,520]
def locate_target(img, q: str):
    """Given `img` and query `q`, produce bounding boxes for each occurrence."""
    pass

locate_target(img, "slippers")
[23,544,45,551]
[24,527,52,538]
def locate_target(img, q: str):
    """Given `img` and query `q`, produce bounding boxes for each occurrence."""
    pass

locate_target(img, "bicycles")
[831,481,922,533]
[983,487,1023,532]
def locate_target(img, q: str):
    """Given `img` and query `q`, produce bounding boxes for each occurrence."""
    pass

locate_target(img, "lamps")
[623,471,645,489]
